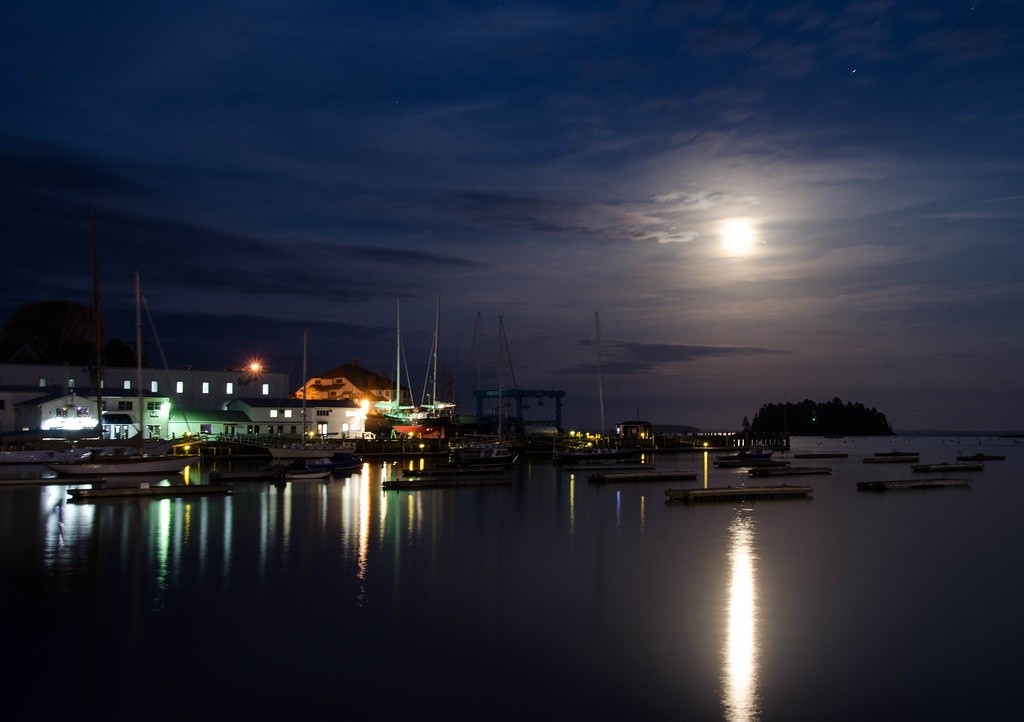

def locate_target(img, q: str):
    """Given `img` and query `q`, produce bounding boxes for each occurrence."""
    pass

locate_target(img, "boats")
[399,463,518,477]
[715,450,773,460]
[862,457,921,463]
[747,467,833,477]
[560,461,658,475]
[824,434,844,438]
[875,452,920,457]
[283,470,332,481]
[855,478,972,493]
[910,462,986,473]
[794,453,849,459]
[66,483,237,500]
[587,471,697,485]
[713,461,792,468]
[665,484,817,506]
[309,459,364,473]
[380,476,514,487]
[956,454,1007,463]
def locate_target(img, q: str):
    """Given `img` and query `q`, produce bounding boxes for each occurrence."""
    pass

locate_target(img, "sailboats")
[43,269,203,476]
[266,321,357,462]
[445,312,526,463]
[556,310,636,464]
[377,286,454,439]
